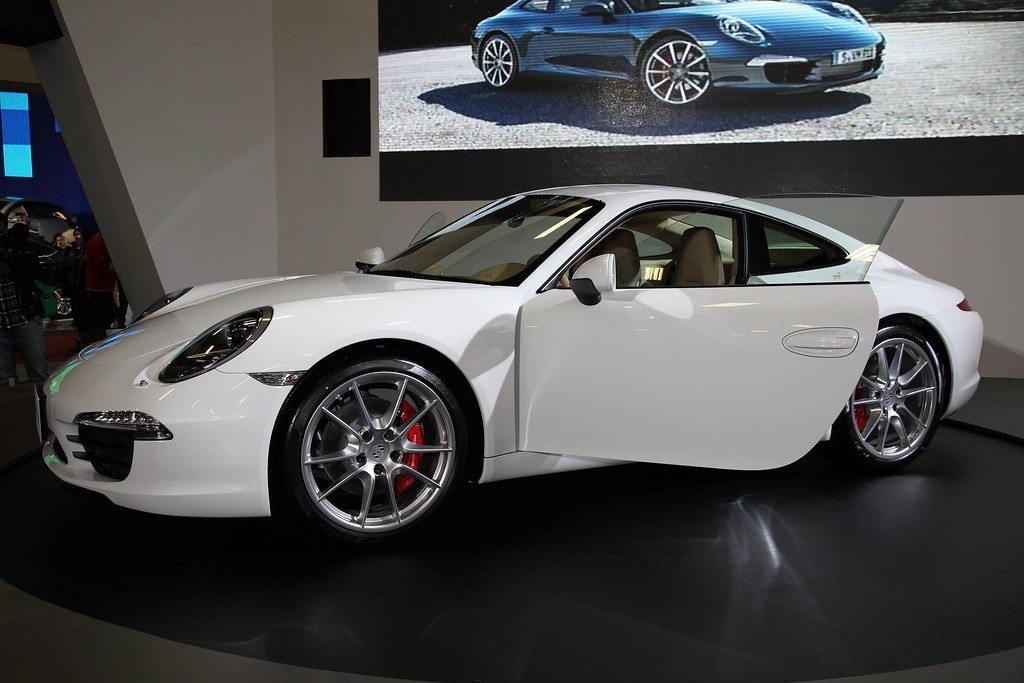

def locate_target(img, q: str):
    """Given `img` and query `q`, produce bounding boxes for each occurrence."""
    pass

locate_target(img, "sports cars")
[43,183,985,554]
[470,0,885,114]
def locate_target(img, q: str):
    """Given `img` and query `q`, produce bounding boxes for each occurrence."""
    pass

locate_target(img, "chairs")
[584,229,640,286]
[664,227,725,287]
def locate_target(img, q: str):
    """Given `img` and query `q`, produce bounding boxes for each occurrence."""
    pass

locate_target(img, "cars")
[0,196,83,319]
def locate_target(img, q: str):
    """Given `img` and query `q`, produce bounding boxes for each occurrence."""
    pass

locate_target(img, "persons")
[0,211,128,387]
